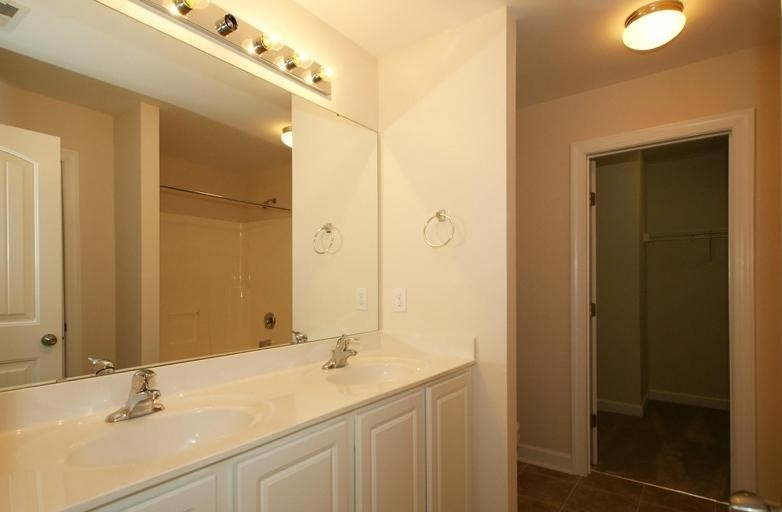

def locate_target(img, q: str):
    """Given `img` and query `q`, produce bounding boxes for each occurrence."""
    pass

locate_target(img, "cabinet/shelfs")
[84,369,349,512]
[351,367,475,510]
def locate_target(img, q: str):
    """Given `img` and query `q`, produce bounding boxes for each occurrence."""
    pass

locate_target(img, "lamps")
[163,1,334,86]
[616,0,689,52]
[280,126,293,148]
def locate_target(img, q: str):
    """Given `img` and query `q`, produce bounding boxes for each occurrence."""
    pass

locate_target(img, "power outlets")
[391,286,407,314]
[353,288,368,313]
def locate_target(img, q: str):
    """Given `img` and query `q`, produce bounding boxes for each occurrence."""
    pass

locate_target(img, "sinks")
[326,358,418,387]
[57,400,259,477]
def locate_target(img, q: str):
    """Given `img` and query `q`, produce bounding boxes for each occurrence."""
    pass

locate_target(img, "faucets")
[321,336,359,370]
[290,329,307,343]
[87,357,116,377]
[105,369,166,422]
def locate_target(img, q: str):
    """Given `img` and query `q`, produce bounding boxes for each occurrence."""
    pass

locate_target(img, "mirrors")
[0,0,382,395]
